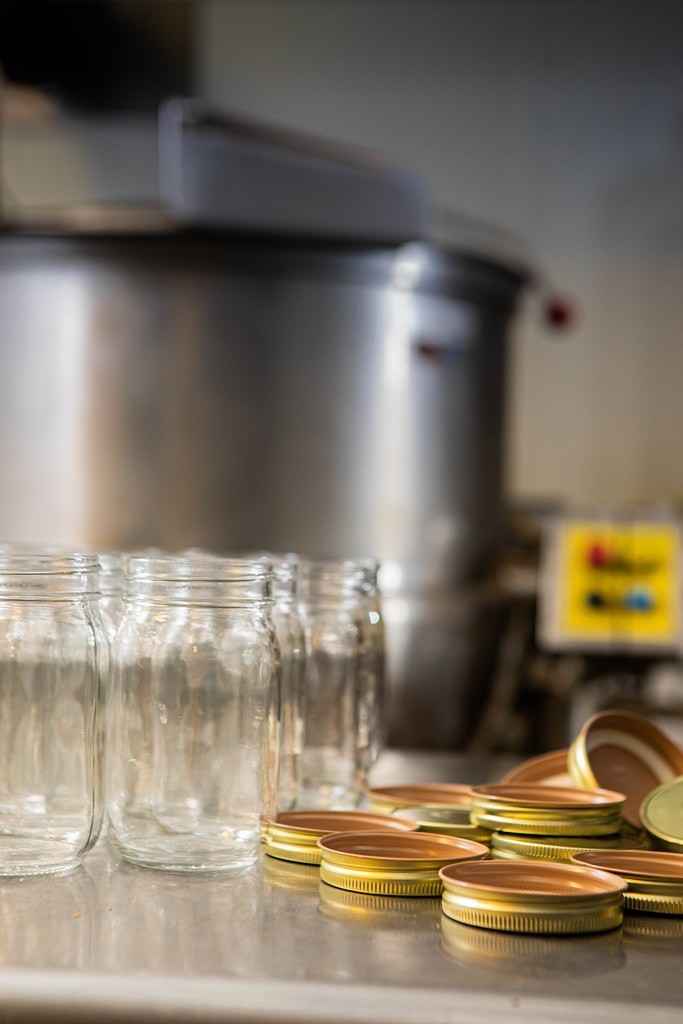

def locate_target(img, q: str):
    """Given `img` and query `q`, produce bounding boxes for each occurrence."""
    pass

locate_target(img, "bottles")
[0,546,389,880]
